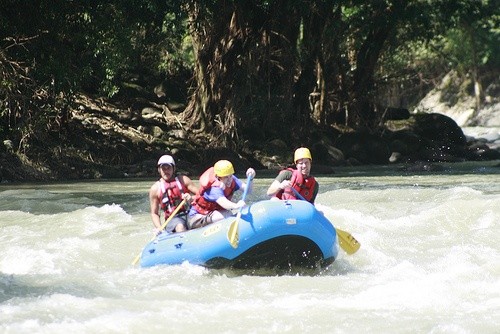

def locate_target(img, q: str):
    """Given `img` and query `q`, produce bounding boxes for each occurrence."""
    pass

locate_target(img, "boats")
[139,196,339,276]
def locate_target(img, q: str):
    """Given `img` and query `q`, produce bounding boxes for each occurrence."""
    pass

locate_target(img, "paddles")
[287,184,361,257]
[133,198,186,266]
[226,172,252,249]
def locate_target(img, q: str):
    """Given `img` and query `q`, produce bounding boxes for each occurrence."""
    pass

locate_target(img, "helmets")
[294,147,312,164]
[158,154,176,166]
[214,159,235,177]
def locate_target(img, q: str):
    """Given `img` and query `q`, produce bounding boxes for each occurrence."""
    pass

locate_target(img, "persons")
[187,160,255,229]
[267,148,319,206]
[149,155,198,234]
